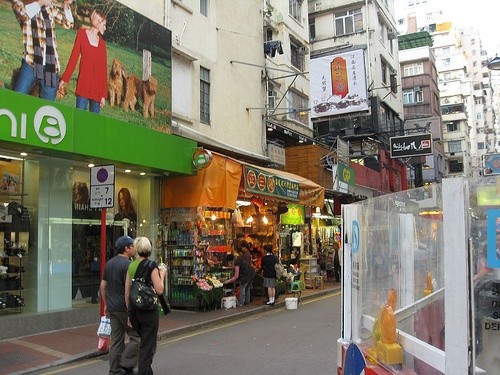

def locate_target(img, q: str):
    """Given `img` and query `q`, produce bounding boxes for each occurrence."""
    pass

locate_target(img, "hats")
[115,236,134,250]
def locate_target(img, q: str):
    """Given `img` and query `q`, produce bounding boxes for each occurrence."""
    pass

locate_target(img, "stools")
[291,282,302,292]
[313,276,324,289]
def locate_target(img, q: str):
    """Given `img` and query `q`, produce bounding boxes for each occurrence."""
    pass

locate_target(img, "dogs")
[108,58,128,107]
[124,73,158,119]
[11,68,67,102]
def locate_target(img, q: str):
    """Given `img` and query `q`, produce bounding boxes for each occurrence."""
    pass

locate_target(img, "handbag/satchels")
[96,314,112,353]
[130,260,161,312]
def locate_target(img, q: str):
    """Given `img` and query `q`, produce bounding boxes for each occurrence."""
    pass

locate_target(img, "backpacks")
[275,263,285,280]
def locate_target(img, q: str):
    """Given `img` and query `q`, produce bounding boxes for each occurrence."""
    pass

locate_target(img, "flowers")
[207,276,223,288]
[196,278,213,292]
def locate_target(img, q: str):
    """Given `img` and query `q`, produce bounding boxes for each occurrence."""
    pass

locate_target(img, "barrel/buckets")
[285,298,298,309]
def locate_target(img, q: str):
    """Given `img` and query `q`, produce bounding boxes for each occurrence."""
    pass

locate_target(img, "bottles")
[168,224,181,245]
[170,266,180,275]
[203,234,212,245]
[180,286,188,302]
[188,287,197,302]
[181,224,198,244]
[214,234,226,246]
[171,258,182,266]
[206,220,224,230]
[171,276,183,285]
[182,258,194,266]
[207,265,233,280]
[172,286,180,301]
[184,276,193,285]
[181,266,193,276]
[182,246,194,256]
[170,246,182,256]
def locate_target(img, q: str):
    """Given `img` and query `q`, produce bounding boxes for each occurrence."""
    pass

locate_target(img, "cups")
[158,263,167,280]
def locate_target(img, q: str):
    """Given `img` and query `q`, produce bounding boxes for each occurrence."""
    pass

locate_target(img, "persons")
[240,242,261,300]
[332,242,342,282]
[124,237,166,375]
[10,0,74,102]
[101,236,141,375]
[259,244,280,305]
[59,9,108,113]
[222,254,257,305]
[112,188,136,238]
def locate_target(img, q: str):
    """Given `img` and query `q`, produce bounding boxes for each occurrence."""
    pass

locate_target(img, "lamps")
[211,213,217,221]
[262,212,268,224]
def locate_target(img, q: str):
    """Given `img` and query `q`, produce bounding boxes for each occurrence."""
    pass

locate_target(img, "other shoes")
[265,302,275,306]
[120,367,134,375]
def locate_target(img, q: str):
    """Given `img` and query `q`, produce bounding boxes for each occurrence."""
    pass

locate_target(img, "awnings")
[162,147,326,209]
[324,188,368,218]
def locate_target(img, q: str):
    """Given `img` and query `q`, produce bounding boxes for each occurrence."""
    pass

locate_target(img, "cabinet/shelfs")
[0,156,30,313]
[169,210,317,310]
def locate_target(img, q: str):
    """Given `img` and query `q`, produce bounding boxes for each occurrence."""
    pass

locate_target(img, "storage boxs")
[222,297,237,309]
